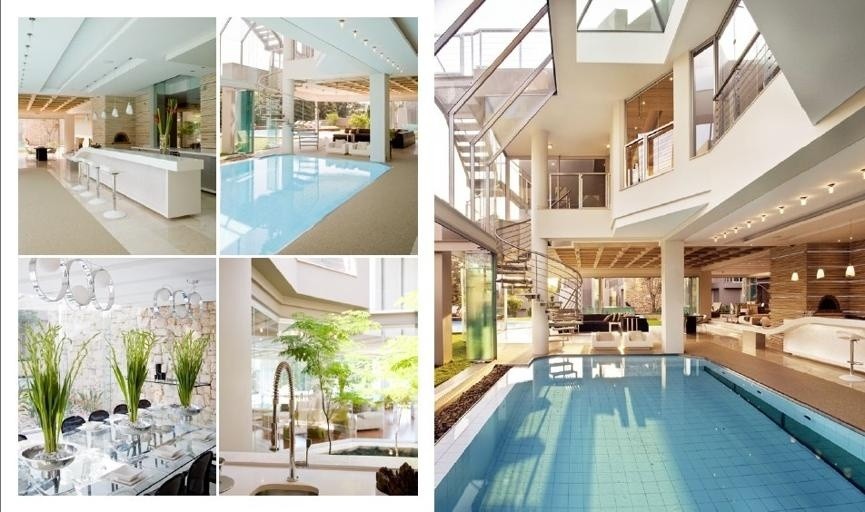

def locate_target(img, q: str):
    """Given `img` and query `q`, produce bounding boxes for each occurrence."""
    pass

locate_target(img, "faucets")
[269,362,301,482]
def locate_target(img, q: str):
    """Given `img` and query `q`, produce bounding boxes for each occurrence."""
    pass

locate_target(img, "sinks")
[250,484,320,496]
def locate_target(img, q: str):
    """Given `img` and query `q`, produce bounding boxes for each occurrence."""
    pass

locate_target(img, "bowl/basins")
[19,441,81,469]
[113,418,152,434]
[191,428,215,439]
[137,408,148,415]
[170,403,205,415]
[219,457,225,483]
[80,421,104,429]
[154,444,183,456]
[112,464,144,481]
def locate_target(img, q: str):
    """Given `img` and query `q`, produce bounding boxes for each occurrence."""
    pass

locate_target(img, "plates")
[75,423,111,432]
[98,471,153,488]
[126,411,153,417]
[151,451,189,462]
[182,432,217,446]
